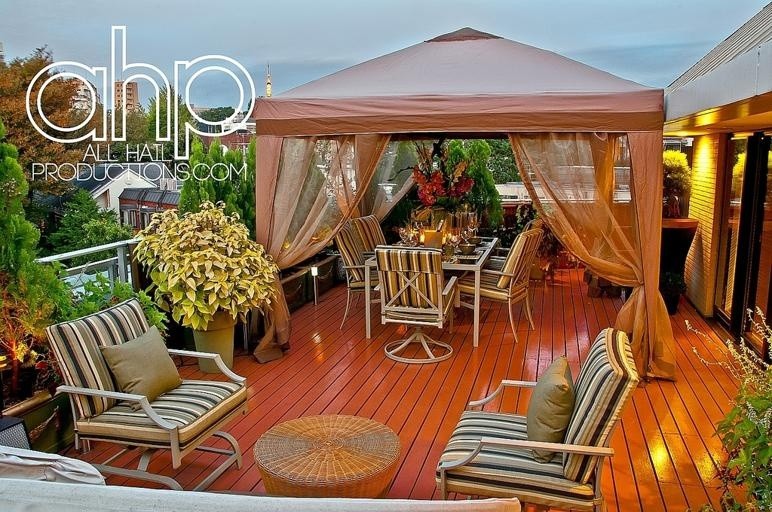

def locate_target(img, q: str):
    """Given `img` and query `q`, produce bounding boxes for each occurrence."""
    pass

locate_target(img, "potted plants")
[133,200,280,374]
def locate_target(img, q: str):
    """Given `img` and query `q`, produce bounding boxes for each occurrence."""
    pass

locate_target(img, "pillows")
[526,356,575,465]
[99,325,182,411]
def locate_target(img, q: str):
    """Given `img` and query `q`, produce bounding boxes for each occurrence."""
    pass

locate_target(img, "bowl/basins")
[468,237,482,244]
[458,244,476,254]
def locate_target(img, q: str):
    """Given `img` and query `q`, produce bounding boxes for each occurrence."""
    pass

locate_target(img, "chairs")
[332,214,544,364]
[46,298,246,492]
[436,328,640,511]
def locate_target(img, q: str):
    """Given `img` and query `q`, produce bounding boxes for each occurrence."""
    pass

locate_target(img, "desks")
[252,414,401,498]
